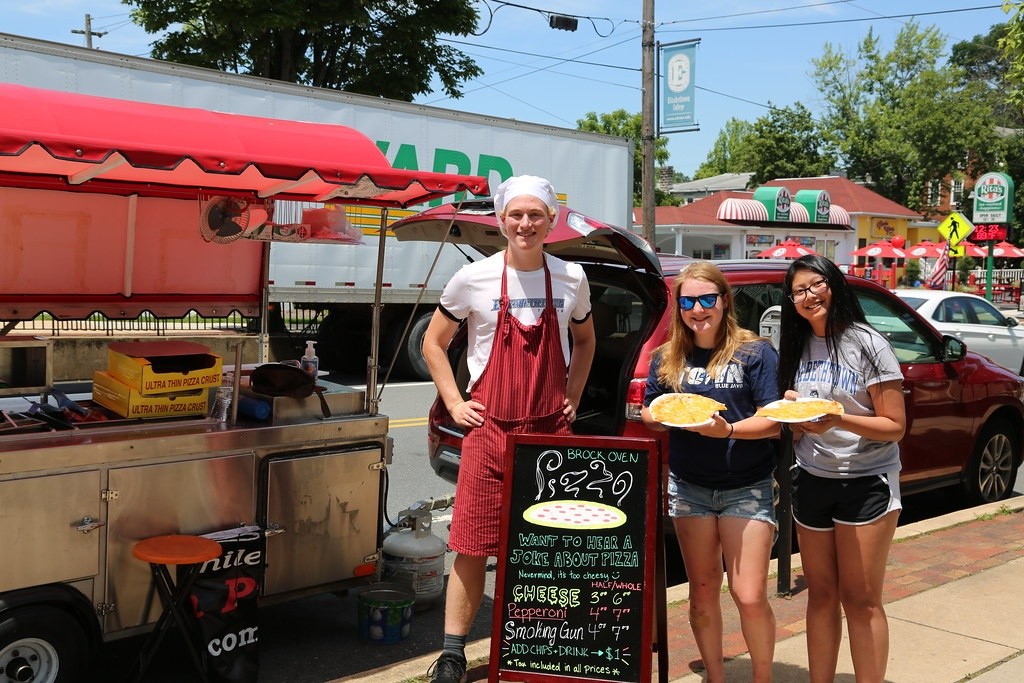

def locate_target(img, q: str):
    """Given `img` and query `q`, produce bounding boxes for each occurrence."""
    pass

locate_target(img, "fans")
[199,194,251,245]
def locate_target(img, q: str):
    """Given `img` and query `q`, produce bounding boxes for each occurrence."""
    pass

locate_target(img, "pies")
[755,400,845,418]
[651,393,728,424]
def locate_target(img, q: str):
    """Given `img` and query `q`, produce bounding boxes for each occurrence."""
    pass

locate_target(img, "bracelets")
[726,424,734,438]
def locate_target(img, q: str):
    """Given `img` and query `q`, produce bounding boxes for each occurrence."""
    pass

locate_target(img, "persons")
[422,173,596,683]
[778,253,905,683]
[638,263,785,683]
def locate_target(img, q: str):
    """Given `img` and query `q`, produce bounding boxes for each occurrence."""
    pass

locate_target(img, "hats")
[494,175,560,239]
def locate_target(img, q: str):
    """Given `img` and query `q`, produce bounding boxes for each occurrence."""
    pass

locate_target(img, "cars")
[759,288,1024,377]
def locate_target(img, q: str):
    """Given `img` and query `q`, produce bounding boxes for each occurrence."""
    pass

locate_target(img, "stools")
[133,536,222,681]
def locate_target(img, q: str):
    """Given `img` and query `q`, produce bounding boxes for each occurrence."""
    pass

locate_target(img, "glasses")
[677,289,727,311]
[788,278,828,304]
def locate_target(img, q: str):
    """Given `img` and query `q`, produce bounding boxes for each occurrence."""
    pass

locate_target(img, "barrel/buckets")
[350,582,417,644]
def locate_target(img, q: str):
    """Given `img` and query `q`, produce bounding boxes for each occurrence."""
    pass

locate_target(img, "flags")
[930,244,948,285]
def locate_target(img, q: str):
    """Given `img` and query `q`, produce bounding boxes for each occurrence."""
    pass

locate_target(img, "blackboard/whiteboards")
[488,433,669,683]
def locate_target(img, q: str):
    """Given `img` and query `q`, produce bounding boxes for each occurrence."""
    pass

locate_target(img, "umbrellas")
[900,242,941,286]
[757,239,822,260]
[849,237,910,266]
[946,238,1024,270]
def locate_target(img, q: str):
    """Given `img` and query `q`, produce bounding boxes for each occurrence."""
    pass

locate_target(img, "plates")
[649,392,719,428]
[764,398,830,423]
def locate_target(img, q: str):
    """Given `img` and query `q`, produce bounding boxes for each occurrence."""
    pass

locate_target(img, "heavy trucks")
[0,32,634,382]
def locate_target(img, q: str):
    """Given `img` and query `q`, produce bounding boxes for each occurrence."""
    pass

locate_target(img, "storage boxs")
[108,342,223,399]
[92,372,208,420]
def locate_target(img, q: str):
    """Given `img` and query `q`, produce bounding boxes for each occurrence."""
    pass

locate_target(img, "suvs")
[376,197,1024,586]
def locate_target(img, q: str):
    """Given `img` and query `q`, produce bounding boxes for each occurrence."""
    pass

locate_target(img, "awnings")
[713,187,856,233]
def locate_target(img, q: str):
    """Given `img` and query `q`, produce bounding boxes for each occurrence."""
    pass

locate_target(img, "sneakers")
[427,651,468,683]
